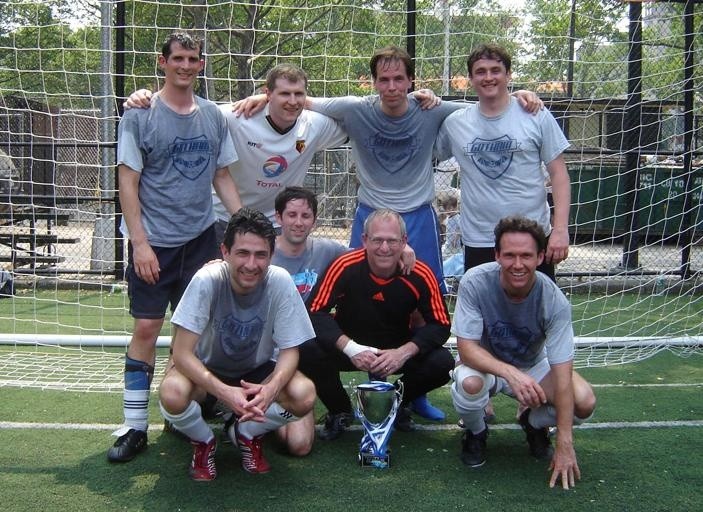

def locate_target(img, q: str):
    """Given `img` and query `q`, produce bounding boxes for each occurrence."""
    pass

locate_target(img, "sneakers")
[409,393,447,421]
[459,424,491,470]
[106,424,149,463]
[392,399,417,434]
[314,410,356,439]
[519,409,554,462]
[227,421,269,474]
[189,436,219,485]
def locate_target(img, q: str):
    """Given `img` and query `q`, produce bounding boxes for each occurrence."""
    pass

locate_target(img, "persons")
[450,215,596,491]
[231,45,545,421]
[158,205,317,482]
[122,61,442,256]
[201,186,416,457]
[297,207,454,439]
[434,44,572,288]
[105,31,243,464]
